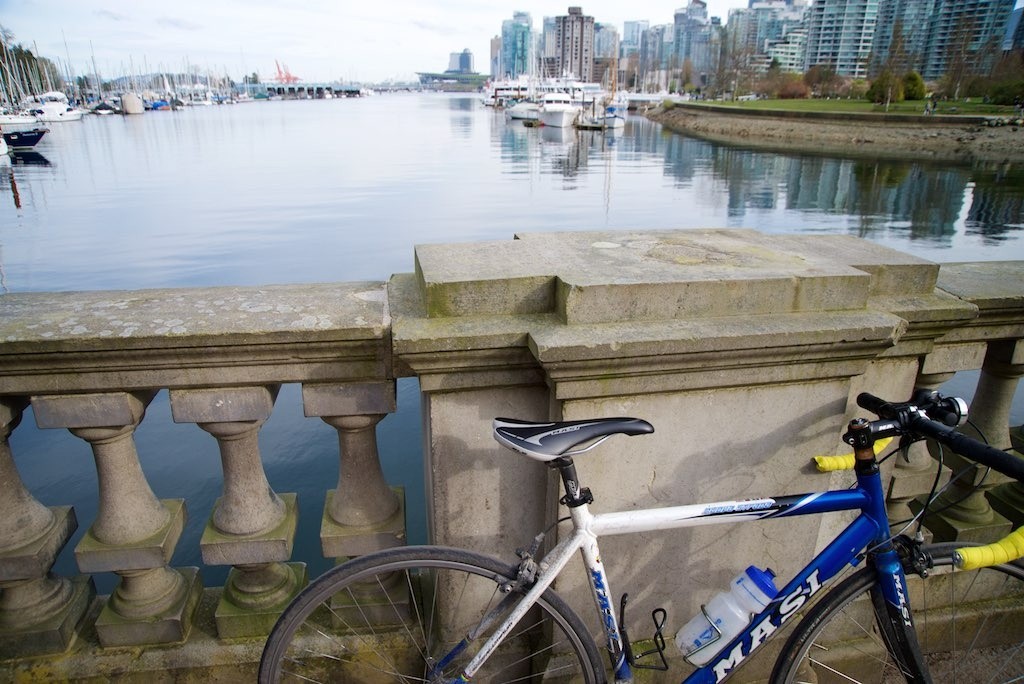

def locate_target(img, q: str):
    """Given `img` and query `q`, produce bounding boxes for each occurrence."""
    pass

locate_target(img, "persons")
[932,99,937,113]
[924,100,931,116]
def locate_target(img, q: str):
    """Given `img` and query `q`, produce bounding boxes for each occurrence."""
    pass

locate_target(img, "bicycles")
[258,386,1023,684]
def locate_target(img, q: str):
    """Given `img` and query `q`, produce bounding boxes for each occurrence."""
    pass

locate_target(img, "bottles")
[673,566,778,665]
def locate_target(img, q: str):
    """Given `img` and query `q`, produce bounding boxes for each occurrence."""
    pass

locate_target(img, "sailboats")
[481,50,626,130]
[0,38,254,155]
